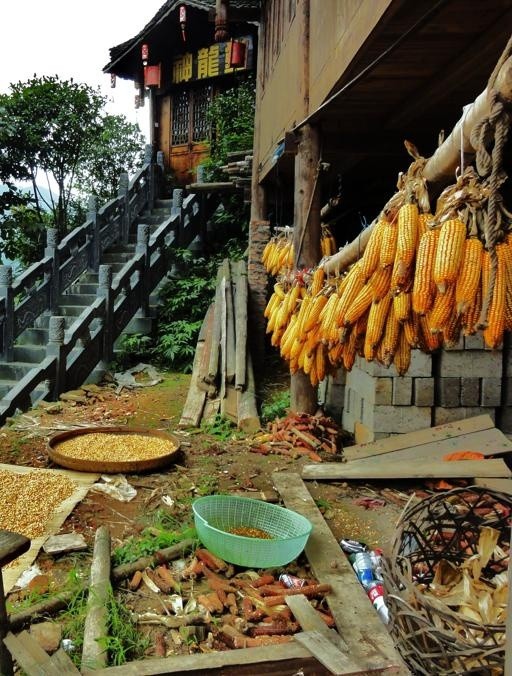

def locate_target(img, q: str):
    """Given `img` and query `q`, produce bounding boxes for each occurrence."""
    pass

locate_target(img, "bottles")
[368,580,389,624]
[339,537,367,554]
[355,553,373,587]
[279,573,305,588]
